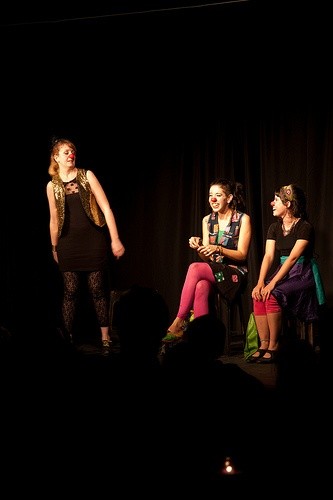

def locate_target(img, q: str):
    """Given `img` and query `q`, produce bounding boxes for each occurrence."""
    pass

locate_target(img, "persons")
[46,137,125,357]
[0,285,333,499]
[245,184,315,365]
[161,178,252,344]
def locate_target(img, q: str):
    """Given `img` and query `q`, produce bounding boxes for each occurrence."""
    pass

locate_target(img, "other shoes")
[255,349,278,364]
[250,349,268,363]
[103,336,112,357]
[163,329,185,343]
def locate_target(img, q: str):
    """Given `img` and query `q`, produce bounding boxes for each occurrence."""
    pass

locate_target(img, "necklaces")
[280,217,297,232]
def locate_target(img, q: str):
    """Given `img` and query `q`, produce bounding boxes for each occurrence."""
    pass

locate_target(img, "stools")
[210,290,245,358]
[282,312,319,352]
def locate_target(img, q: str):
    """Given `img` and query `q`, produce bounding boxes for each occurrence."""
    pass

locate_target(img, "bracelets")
[50,244,57,253]
[219,246,222,255]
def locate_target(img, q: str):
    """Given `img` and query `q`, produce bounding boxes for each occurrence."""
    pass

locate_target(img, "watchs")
[215,245,219,253]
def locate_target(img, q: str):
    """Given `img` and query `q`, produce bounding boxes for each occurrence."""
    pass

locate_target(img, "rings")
[262,292,265,295]
